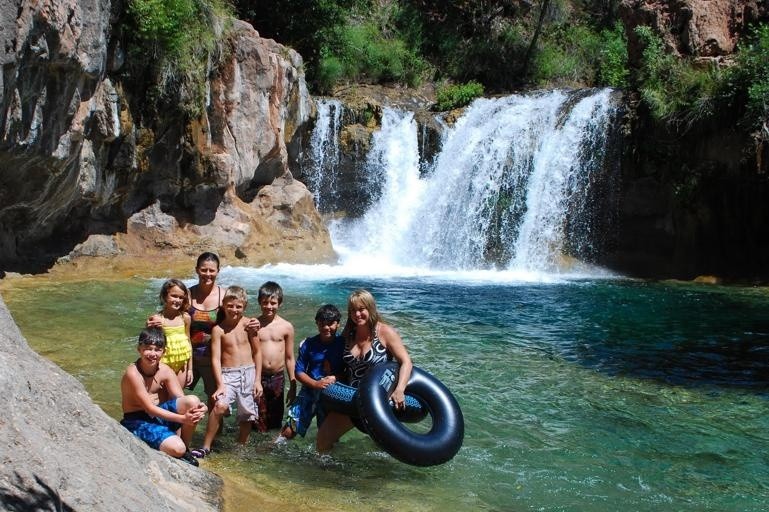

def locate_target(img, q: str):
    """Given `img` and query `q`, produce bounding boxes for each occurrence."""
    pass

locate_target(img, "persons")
[150,279,196,404]
[189,285,263,458]
[120,323,210,467]
[145,251,262,437]
[253,281,298,433]
[315,288,413,456]
[279,304,345,440]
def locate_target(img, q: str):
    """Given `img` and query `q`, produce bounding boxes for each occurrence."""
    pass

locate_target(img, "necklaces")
[136,357,160,377]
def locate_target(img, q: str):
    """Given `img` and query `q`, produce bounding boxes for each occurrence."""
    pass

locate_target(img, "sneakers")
[192,446,209,457]
[181,449,199,466]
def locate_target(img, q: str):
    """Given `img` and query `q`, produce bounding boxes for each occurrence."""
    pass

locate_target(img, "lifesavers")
[360,361,464,467]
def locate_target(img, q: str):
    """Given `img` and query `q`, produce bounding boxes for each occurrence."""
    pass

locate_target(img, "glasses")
[139,338,165,348]
[317,311,337,321]
